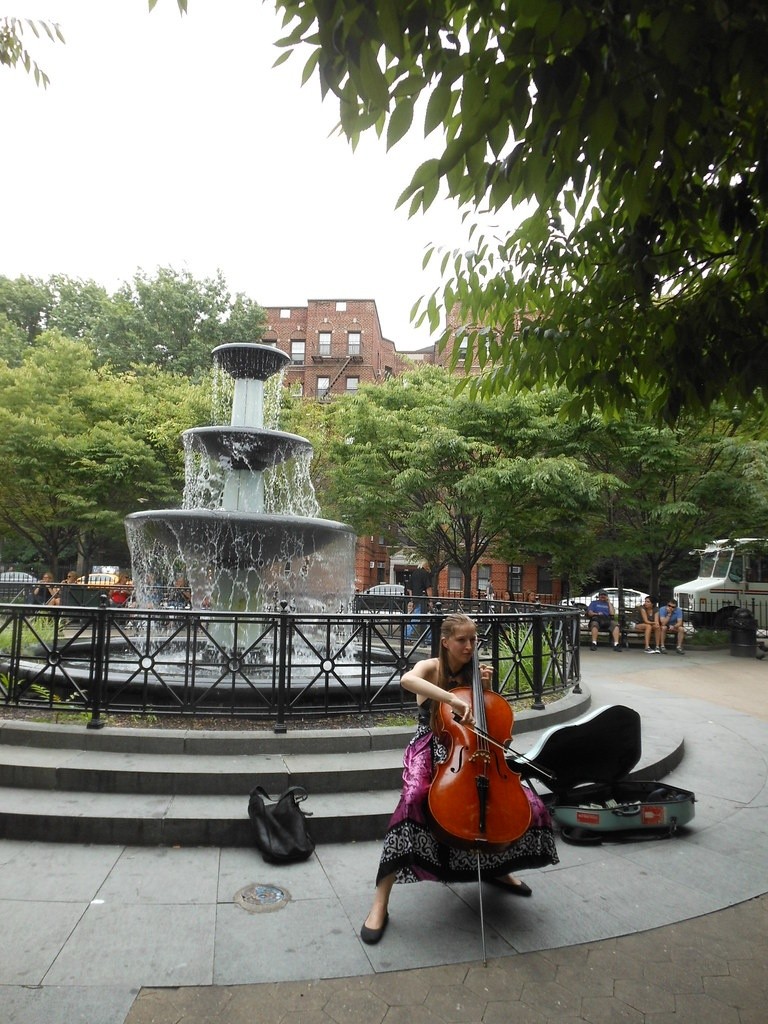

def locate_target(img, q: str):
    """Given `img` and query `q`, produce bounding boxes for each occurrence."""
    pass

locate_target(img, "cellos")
[424,634,532,967]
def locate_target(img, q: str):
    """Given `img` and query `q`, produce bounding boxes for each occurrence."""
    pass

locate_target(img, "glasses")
[668,604,676,608]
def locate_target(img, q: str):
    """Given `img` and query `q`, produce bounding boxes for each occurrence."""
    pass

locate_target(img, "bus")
[672,537,768,633]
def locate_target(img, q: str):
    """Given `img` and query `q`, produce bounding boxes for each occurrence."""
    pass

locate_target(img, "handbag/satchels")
[248,786,315,864]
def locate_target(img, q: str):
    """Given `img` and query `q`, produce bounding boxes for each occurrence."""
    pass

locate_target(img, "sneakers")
[613,644,622,652]
[654,647,661,654]
[590,642,598,651]
[644,646,655,654]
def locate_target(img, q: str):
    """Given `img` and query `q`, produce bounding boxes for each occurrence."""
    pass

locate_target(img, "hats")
[599,590,608,595]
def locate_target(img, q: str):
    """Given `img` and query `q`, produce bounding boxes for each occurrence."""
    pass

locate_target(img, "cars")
[557,587,651,616]
[355,582,411,610]
[0,572,41,589]
[66,573,134,589]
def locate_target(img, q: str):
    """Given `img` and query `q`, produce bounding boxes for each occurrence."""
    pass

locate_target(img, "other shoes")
[489,877,532,896]
[360,911,389,944]
[675,648,685,654]
[660,646,668,654]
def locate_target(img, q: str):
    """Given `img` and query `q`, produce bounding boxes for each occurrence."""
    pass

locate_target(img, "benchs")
[573,603,614,647]
[619,607,678,649]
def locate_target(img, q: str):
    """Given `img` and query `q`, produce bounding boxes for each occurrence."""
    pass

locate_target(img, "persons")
[359,613,560,946]
[397,561,499,647]
[658,598,687,654]
[585,589,623,652]
[23,566,209,629]
[635,595,662,655]
[501,590,522,644]
[521,590,542,618]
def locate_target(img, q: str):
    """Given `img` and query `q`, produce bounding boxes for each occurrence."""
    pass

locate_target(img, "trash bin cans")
[727,608,759,658]
